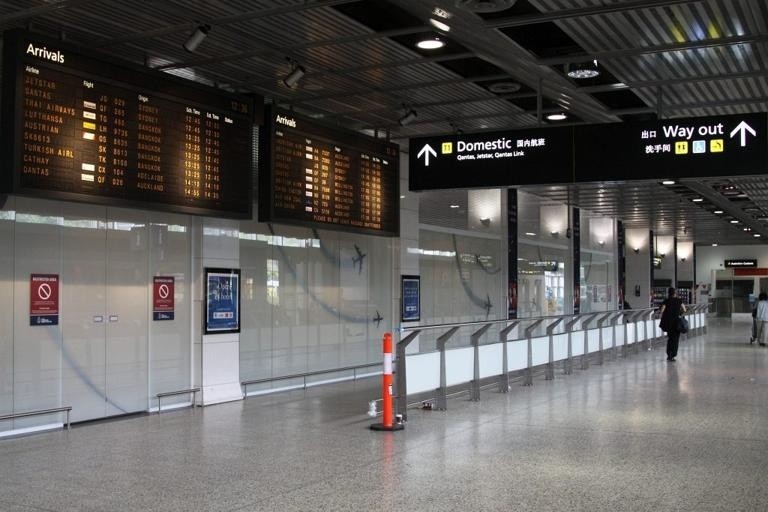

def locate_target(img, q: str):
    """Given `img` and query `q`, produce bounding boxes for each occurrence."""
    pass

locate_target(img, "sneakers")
[667,357,676,361]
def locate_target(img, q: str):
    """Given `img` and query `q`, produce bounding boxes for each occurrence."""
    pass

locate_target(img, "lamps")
[181,20,211,53]
[398,103,418,127]
[282,59,306,88]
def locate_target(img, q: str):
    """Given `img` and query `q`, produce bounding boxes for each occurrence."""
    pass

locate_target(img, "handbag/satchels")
[678,317,688,334]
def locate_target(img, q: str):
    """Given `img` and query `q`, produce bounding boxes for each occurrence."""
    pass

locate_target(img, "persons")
[659,287,687,361]
[752,292,768,345]
[619,293,632,323]
